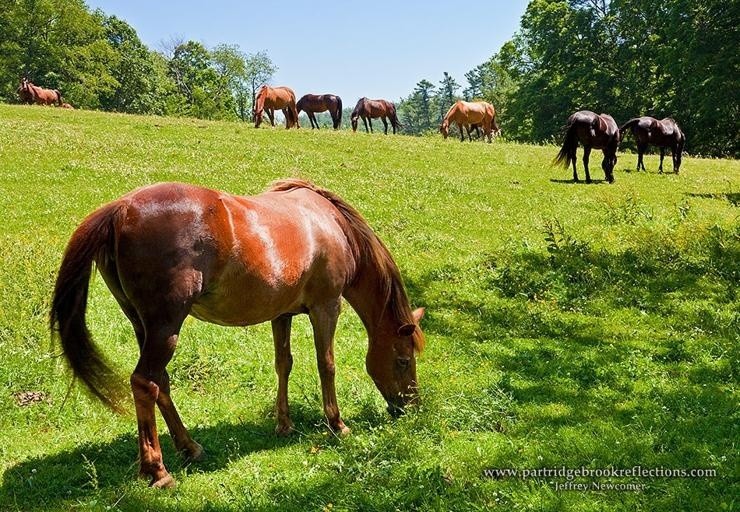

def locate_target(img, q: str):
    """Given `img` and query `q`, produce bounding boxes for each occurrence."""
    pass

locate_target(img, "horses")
[16,75,62,106]
[350,96,401,135]
[285,94,344,130]
[45,178,426,491]
[251,84,301,132]
[437,99,497,144]
[625,115,688,174]
[549,107,622,184]
